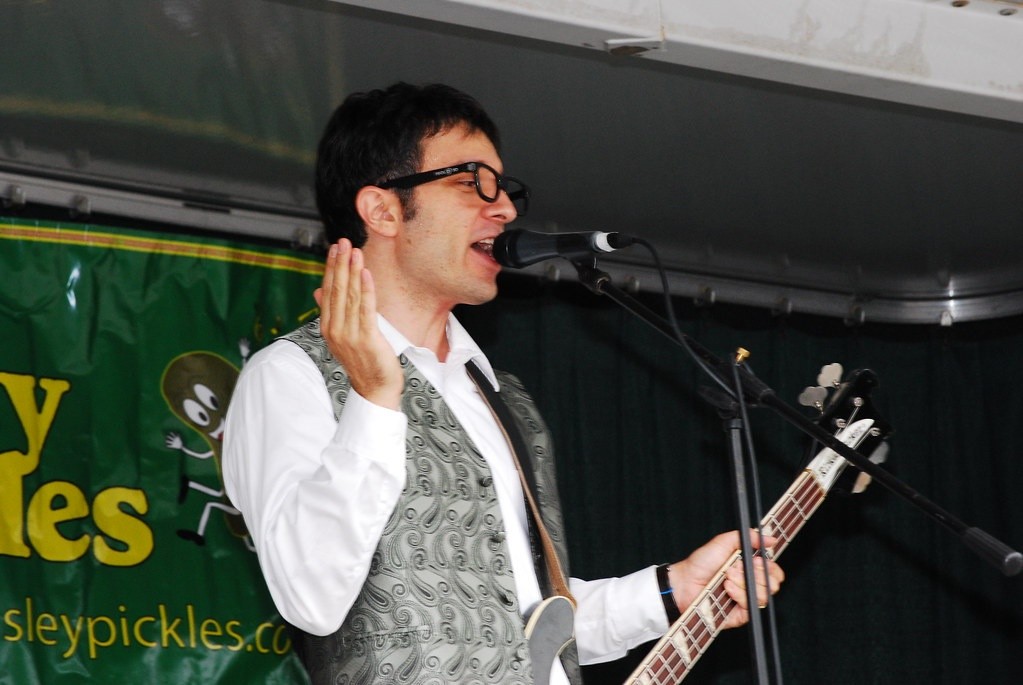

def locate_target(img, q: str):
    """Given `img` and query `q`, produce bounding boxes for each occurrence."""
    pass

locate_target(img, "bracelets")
[656,563,683,624]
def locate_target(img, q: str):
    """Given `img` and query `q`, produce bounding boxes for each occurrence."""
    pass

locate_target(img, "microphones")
[492,226,636,270]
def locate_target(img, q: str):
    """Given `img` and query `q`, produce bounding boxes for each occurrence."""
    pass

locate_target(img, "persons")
[220,79,785,685]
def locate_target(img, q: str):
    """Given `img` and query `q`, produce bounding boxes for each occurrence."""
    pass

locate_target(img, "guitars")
[523,360,894,685]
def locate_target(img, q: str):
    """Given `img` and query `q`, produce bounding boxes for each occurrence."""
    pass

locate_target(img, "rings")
[758,605,766,609]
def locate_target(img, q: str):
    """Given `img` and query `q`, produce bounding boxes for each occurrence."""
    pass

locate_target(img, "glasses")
[376,162,531,216]
